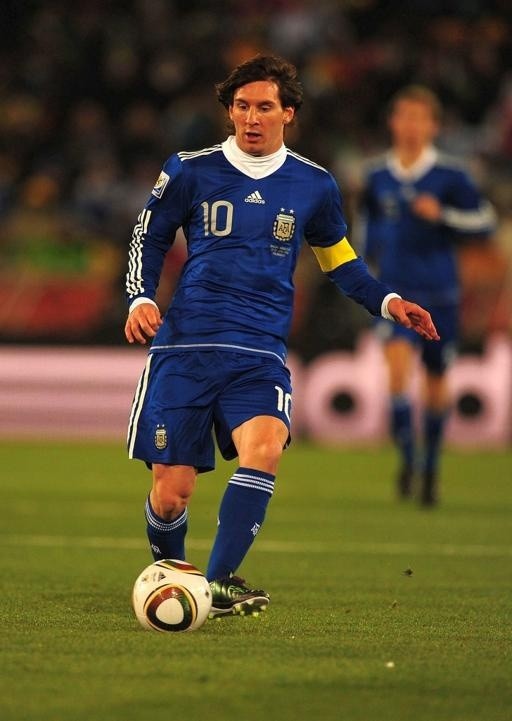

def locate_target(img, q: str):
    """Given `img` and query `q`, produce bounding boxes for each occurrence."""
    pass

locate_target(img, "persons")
[1,2,511,360]
[347,81,497,511]
[122,53,440,621]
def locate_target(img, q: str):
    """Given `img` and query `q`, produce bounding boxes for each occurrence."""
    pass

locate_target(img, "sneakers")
[207,574,271,621]
[397,459,441,506]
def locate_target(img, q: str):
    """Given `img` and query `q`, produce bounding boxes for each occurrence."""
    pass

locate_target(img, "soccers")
[132,558,213,635]
